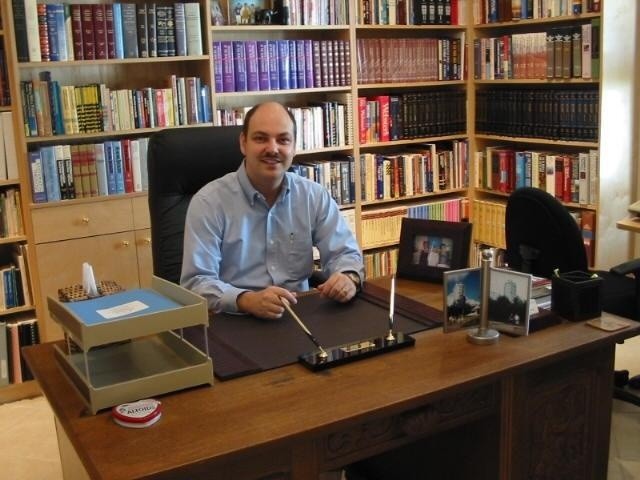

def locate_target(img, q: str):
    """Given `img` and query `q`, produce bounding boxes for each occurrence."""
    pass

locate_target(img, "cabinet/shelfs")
[0,0,469,405]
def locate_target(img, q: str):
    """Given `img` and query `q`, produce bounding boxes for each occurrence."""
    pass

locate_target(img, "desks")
[19,273,640,479]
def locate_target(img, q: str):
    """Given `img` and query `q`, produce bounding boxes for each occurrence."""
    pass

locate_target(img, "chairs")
[147,125,246,287]
[505,186,640,387]
[470,0,637,278]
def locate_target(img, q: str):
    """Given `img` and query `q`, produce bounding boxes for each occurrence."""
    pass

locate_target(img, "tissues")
[59,262,132,356]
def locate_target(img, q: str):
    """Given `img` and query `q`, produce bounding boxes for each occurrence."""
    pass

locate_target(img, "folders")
[63,287,185,324]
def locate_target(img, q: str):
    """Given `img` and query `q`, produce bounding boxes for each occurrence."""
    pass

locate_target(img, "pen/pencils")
[389,276,396,328]
[295,290,322,298]
[278,296,325,353]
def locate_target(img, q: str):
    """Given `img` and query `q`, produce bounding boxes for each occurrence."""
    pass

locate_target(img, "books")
[580,210,595,265]
[216,102,255,128]
[361,196,470,249]
[355,39,463,85]
[359,140,470,202]
[2,111,26,240]
[289,155,357,206]
[1,317,40,388]
[475,88,599,143]
[27,137,148,204]
[19,70,213,138]
[471,245,503,268]
[0,244,33,312]
[11,0,203,62]
[288,93,354,153]
[358,90,466,145]
[479,0,601,23]
[363,247,399,281]
[474,146,598,206]
[213,39,353,94]
[474,19,600,79]
[211,1,460,25]
[472,199,506,250]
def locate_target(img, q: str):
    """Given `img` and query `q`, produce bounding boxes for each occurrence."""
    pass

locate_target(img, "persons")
[428,246,439,266]
[446,283,474,321]
[419,241,428,266]
[439,244,449,264]
[492,281,527,327]
[412,245,419,264]
[180,101,365,320]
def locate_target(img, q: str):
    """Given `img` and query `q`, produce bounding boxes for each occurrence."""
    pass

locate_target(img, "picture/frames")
[395,217,474,285]
[441,266,482,334]
[486,265,532,338]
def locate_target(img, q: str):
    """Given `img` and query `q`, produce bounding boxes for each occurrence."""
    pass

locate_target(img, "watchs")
[340,271,363,288]
